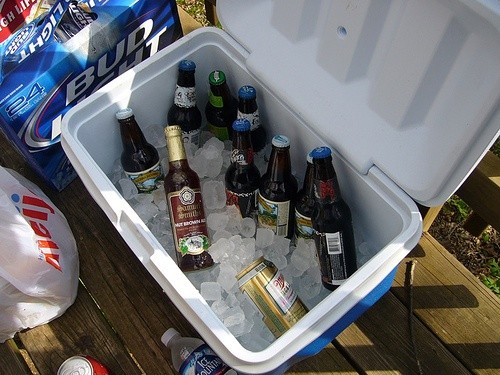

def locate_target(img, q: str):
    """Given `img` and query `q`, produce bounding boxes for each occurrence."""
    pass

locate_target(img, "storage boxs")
[0,0,185,195]
[0,0,51,44]
[58,0,500,375]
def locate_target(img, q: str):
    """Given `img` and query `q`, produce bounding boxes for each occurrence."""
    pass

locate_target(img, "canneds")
[235,255,311,338]
[57,353,113,375]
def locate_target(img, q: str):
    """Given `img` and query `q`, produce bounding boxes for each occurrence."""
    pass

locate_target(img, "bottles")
[294,151,315,247]
[258,135,297,243]
[312,147,358,291]
[237,86,265,151]
[162,126,214,273]
[166,60,203,149]
[204,70,236,142]
[224,121,257,218]
[114,108,166,211]
[161,328,238,375]
